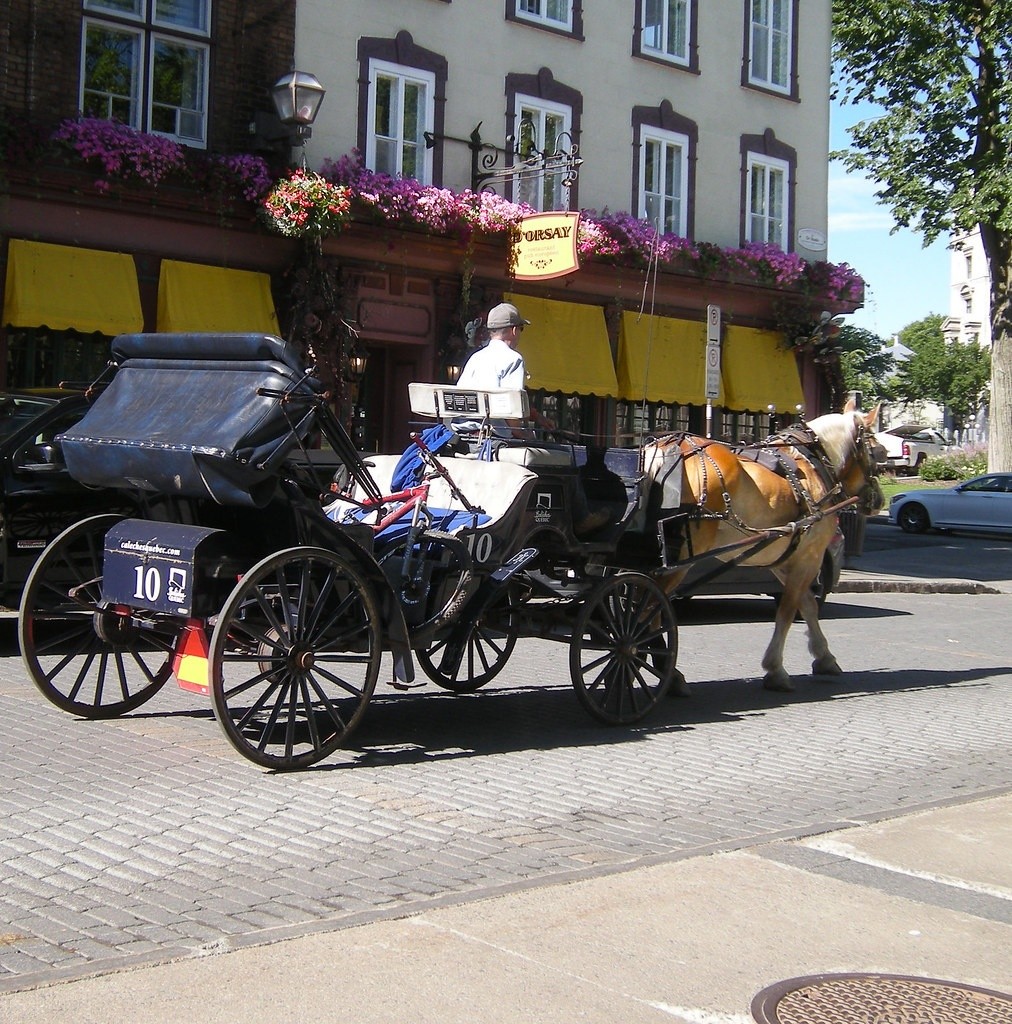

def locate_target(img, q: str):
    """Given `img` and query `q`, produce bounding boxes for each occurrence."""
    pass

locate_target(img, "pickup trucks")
[875,425,961,476]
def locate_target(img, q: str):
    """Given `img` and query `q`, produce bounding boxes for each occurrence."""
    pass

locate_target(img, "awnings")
[4,237,145,338]
[502,287,806,416]
[155,257,283,340]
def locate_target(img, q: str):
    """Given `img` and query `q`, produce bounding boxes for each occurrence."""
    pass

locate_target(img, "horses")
[638,398,886,693]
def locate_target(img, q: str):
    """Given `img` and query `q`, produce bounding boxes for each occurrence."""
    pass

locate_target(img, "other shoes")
[573,503,616,535]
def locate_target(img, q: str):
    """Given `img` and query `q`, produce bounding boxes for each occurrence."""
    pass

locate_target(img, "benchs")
[336,453,540,567]
[407,383,588,524]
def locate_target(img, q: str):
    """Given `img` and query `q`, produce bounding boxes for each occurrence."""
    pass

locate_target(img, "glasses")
[517,324,525,332]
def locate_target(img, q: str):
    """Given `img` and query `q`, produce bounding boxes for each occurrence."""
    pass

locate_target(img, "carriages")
[18,333,881,771]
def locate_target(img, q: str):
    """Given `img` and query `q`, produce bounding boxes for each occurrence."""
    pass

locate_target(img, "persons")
[457,303,612,537]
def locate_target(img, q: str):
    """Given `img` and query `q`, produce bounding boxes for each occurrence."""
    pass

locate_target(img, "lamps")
[269,69,326,148]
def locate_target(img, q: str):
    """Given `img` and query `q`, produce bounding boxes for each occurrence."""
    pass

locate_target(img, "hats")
[486,302,531,329]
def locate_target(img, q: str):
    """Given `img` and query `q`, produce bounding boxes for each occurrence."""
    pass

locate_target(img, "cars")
[674,526,844,621]
[888,472,1012,534]
[0,388,380,612]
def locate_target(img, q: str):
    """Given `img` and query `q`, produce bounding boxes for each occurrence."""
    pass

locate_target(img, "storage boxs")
[103,518,233,620]
